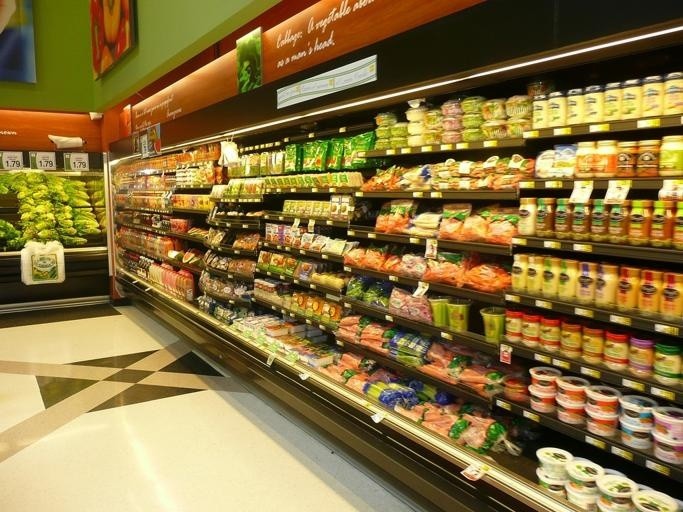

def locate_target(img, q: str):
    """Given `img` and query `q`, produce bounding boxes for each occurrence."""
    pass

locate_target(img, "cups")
[429,296,446,327]
[446,303,470,335]
[481,307,505,347]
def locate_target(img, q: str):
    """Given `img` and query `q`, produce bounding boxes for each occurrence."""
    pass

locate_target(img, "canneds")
[660,272,683,322]
[505,311,522,333]
[642,76,663,116]
[560,321,581,349]
[649,201,673,248]
[663,72,683,116]
[637,270,660,317]
[505,332,521,345]
[527,257,543,298]
[628,337,652,367]
[596,264,618,309]
[576,262,597,306]
[532,95,548,128]
[559,260,578,302]
[593,141,617,178]
[560,345,583,361]
[536,199,555,236]
[652,370,683,388]
[630,362,652,380]
[622,80,642,119]
[603,332,628,361]
[574,141,595,178]
[603,355,628,374]
[583,86,603,123]
[521,314,539,337]
[591,199,607,242]
[630,200,651,244]
[554,199,573,239]
[566,89,583,123]
[607,200,629,245]
[547,92,566,127]
[603,82,619,122]
[572,199,590,242]
[582,328,604,354]
[582,350,604,367]
[636,140,660,177]
[538,319,560,341]
[518,198,537,236]
[511,254,526,294]
[522,334,538,350]
[538,337,560,356]
[617,142,637,176]
[673,202,683,250]
[615,266,639,315]
[658,136,683,176]
[543,258,560,301]
[652,344,681,377]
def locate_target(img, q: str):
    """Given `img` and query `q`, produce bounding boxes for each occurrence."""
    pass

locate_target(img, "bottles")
[511,254,680,325]
[518,199,683,247]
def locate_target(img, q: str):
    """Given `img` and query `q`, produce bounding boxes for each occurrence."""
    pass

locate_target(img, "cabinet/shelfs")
[107,18,683,512]
[0,168,113,314]
